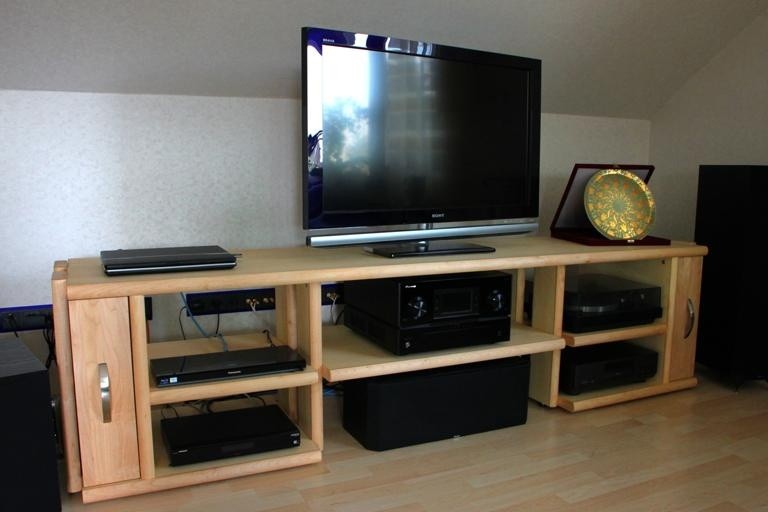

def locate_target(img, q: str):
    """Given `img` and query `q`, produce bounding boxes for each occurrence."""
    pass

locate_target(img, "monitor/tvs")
[303,24,542,260]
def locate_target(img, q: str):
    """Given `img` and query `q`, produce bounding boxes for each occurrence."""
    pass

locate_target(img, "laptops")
[100,244,238,276]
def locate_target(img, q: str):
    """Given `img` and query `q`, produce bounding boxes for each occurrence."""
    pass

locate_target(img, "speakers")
[0,329,63,512]
[344,358,541,449]
[694,167,768,395]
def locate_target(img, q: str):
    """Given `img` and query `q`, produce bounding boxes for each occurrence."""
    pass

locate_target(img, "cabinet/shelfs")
[51,233,708,505]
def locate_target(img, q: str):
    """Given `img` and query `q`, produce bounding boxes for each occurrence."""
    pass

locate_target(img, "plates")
[583,169,655,241]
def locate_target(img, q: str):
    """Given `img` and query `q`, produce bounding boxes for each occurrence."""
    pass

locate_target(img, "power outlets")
[186,283,344,316]
